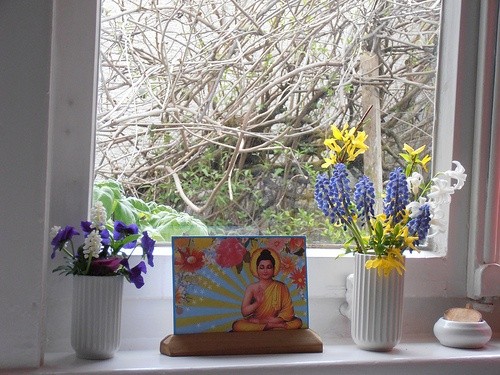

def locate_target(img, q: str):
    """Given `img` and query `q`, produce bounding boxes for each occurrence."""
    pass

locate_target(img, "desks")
[34,334,500,375]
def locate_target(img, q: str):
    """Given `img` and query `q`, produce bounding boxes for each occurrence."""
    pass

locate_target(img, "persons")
[232,250,302,331]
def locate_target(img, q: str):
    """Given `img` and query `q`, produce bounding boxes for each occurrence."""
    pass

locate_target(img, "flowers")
[313,123,469,280]
[49,200,156,291]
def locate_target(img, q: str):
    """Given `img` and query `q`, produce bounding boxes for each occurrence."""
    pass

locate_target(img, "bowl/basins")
[433,318,493,348]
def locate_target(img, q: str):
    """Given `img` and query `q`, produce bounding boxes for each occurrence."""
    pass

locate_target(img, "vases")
[69,273,123,361]
[350,249,405,353]
